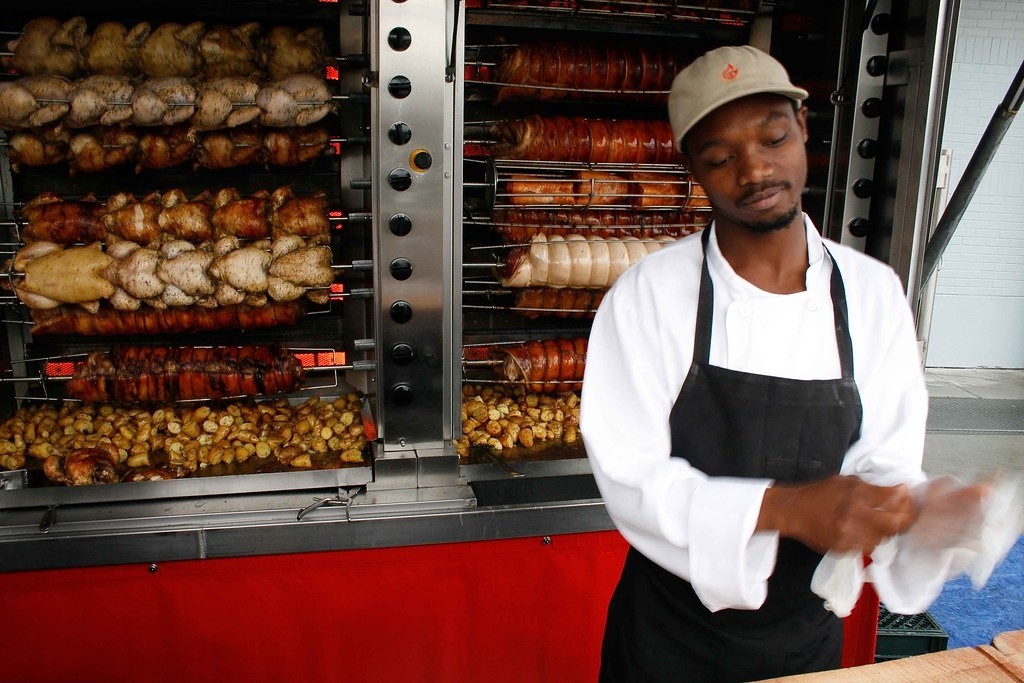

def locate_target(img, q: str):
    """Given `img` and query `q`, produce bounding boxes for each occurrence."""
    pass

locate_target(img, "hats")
[669,45,809,153]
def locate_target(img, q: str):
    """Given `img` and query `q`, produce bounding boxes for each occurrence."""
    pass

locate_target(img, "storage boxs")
[875,604,951,665]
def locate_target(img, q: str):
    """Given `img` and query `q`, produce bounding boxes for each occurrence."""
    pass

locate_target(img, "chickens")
[0,21,338,312]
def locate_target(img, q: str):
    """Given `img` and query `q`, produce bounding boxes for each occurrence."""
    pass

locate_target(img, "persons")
[578,45,1001,683]
